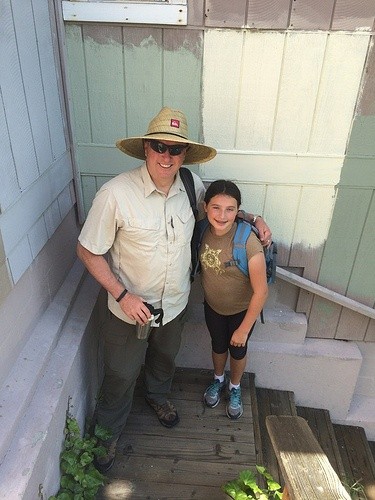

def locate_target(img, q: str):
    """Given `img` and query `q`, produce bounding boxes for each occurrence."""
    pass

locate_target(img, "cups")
[136,314,155,340]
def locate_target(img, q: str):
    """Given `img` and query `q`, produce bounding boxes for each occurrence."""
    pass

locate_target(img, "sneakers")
[225,380,244,419]
[144,396,180,429]
[203,371,228,408]
[92,431,120,472]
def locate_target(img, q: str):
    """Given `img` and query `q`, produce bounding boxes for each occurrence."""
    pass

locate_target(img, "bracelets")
[116,289,128,303]
[252,215,262,225]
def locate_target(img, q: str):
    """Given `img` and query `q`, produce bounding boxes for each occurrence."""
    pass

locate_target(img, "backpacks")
[190,218,277,285]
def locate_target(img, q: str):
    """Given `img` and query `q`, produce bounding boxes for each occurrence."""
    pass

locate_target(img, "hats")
[115,107,217,165]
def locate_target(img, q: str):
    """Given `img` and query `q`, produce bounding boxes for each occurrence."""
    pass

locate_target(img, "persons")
[192,180,270,420]
[75,108,272,474]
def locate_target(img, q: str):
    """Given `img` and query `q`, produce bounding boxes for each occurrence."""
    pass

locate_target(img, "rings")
[268,240,270,242]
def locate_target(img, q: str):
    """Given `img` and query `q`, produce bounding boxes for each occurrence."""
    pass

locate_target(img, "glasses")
[144,139,190,157]
